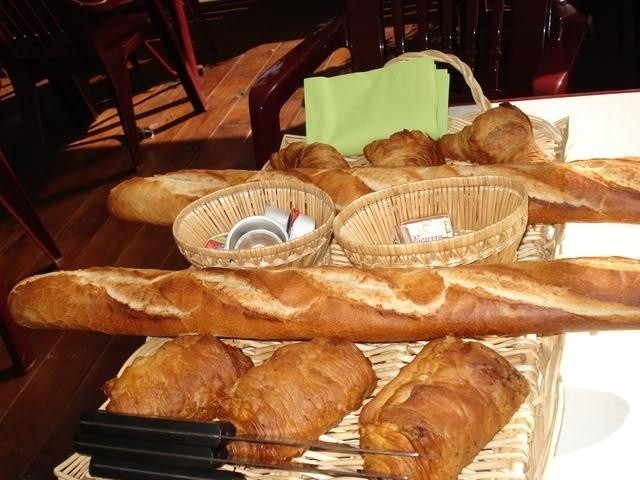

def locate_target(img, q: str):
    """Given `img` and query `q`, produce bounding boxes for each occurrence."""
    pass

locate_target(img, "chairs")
[247,0,589,171]
[0,1,210,378]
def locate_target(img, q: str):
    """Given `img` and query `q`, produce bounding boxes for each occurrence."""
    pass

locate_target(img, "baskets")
[172,180,336,270]
[333,176,529,268]
[52,49,568,480]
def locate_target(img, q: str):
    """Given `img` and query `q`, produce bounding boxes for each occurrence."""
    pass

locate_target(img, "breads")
[106,101,640,224]
[104,334,533,480]
[7,256,640,342]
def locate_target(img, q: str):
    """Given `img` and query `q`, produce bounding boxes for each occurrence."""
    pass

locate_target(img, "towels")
[299,54,451,160]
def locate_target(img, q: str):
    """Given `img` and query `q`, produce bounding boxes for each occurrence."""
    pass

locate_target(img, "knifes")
[80,411,420,460]
[77,440,401,480]
[89,455,308,480]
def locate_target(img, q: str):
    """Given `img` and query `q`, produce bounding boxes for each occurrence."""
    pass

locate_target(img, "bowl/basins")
[205,203,316,256]
